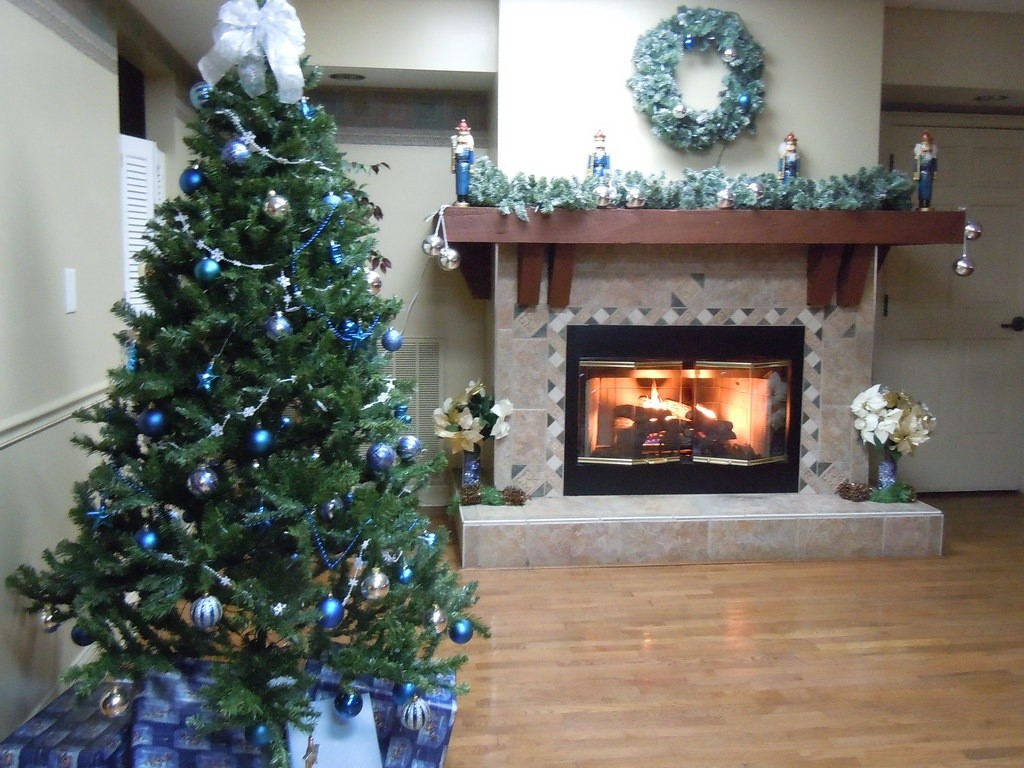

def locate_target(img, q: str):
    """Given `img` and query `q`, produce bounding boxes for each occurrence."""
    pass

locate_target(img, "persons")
[778,132,800,186]
[912,130,939,209]
[587,128,611,183]
[449,118,476,207]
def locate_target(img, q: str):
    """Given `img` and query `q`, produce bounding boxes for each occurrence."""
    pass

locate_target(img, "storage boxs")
[1,642,459,768]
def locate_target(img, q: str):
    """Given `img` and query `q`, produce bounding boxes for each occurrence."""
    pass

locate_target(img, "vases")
[876,444,903,503]
[461,436,480,501]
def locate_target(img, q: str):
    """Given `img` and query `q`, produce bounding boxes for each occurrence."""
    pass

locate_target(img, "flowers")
[845,380,937,455]
[432,380,513,457]
